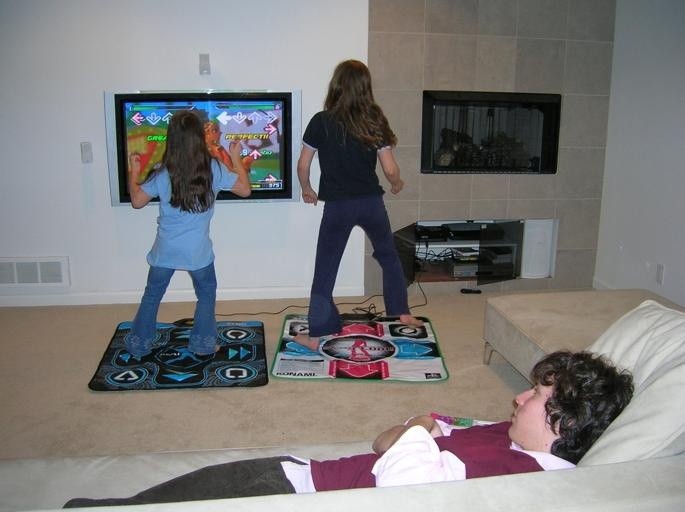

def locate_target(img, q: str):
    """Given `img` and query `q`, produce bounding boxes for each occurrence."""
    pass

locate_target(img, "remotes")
[461,289,481,294]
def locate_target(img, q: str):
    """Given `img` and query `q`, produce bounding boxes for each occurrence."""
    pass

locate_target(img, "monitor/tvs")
[103,90,302,208]
[421,90,561,176]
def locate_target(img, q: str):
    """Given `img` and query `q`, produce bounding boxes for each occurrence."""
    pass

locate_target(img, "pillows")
[576,298,684,464]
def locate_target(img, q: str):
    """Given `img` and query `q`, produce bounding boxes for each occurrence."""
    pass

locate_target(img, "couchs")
[484,288,682,390]
[2,298,683,510]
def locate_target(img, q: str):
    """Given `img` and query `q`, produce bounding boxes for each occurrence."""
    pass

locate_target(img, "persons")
[125,109,252,358]
[62,351,633,510]
[293,58,423,349]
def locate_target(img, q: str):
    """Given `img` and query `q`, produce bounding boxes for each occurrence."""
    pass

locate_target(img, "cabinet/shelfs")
[416,220,524,282]
